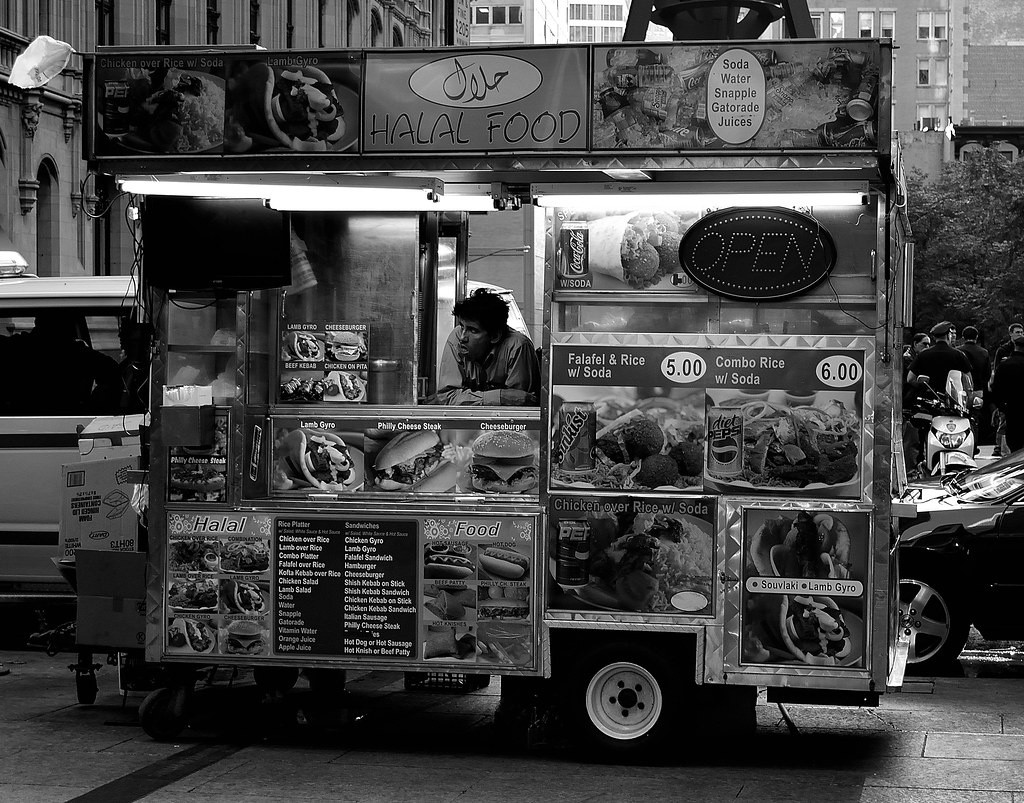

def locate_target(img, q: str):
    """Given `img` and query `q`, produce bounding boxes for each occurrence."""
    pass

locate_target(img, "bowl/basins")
[204,554,218,565]
[783,390,816,406]
[739,388,771,401]
[596,397,636,423]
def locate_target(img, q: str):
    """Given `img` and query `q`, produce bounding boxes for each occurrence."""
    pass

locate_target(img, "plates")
[168,577,218,611]
[455,448,538,495]
[748,605,867,665]
[370,453,456,491]
[218,539,270,574]
[170,538,217,575]
[219,586,271,616]
[284,338,325,362]
[218,629,269,656]
[322,377,365,402]
[549,509,712,612]
[96,68,225,155]
[168,616,215,653]
[271,443,365,493]
[228,80,359,152]
[705,451,860,489]
[422,641,477,662]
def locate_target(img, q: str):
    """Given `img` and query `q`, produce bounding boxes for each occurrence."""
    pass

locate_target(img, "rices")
[581,503,713,612]
[123,68,225,151]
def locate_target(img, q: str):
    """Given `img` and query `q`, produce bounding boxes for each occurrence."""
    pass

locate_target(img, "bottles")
[594,86,604,125]
[621,89,682,120]
[595,66,675,87]
[606,49,663,68]
[599,86,640,142]
[768,72,811,120]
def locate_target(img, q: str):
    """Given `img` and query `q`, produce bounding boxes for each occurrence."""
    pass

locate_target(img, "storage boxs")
[76,414,145,461]
[73,547,147,650]
[56,454,141,564]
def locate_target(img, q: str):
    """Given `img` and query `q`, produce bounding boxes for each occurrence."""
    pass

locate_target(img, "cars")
[0,248,165,607]
[894,446,1024,678]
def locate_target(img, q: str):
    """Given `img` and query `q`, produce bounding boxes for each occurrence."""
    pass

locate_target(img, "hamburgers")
[374,428,451,490]
[229,619,263,654]
[331,332,362,361]
[468,430,538,492]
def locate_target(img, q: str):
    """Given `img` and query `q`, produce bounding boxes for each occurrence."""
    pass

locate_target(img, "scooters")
[909,374,985,479]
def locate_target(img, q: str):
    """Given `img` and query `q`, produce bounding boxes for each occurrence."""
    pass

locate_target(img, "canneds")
[554,518,592,589]
[559,220,589,279]
[102,78,131,137]
[708,406,744,476]
[593,48,878,148]
[557,400,599,474]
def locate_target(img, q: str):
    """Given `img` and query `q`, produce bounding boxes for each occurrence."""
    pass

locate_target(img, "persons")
[900,318,1023,479]
[434,287,540,406]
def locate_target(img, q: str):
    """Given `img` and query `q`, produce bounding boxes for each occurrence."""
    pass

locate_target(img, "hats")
[929,320,952,336]
[1013,336,1024,346]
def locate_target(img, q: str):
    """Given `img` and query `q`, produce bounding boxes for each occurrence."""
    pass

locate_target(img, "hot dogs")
[482,546,529,579]
[425,554,475,577]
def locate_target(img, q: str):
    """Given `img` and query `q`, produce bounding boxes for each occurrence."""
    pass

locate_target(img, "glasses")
[919,341,931,347]
